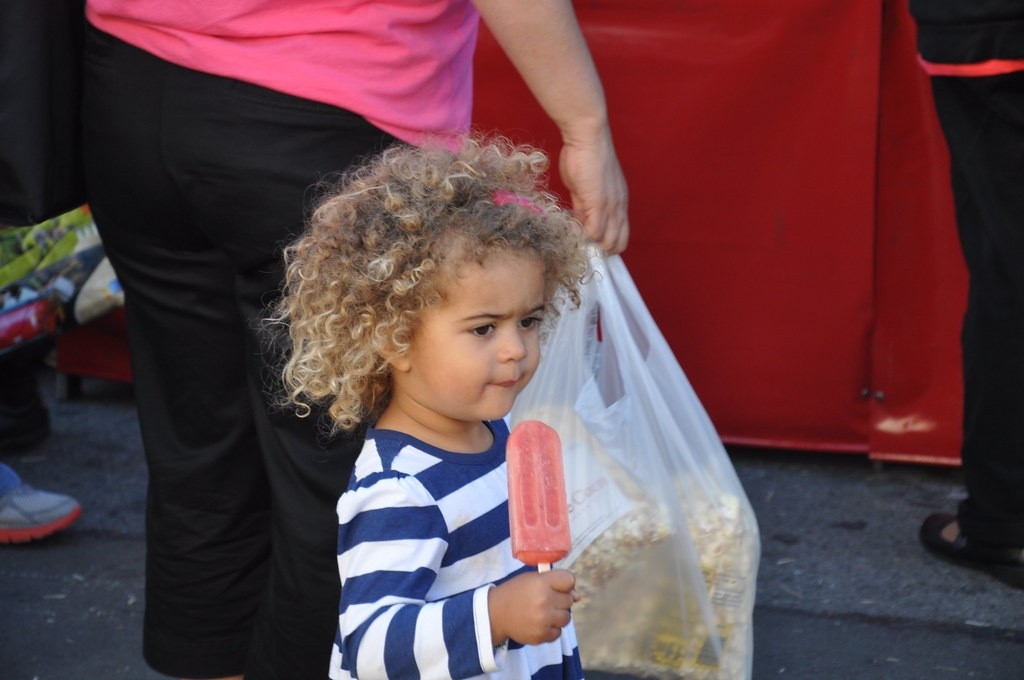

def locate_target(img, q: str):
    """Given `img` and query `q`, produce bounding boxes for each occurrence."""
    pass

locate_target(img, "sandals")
[919,513,1024,590]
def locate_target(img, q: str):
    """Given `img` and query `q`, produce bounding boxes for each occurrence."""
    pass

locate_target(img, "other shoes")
[0,401,50,452]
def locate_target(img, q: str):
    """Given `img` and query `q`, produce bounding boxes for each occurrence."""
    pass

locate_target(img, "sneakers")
[0,462,82,544]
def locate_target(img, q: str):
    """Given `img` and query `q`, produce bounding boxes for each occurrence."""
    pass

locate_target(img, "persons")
[909,1,1024,593]
[3,178,128,548]
[69,0,632,679]
[270,139,587,679]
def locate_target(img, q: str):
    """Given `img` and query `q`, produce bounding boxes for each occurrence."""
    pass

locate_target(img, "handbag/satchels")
[0,0,91,226]
[501,245,761,680]
